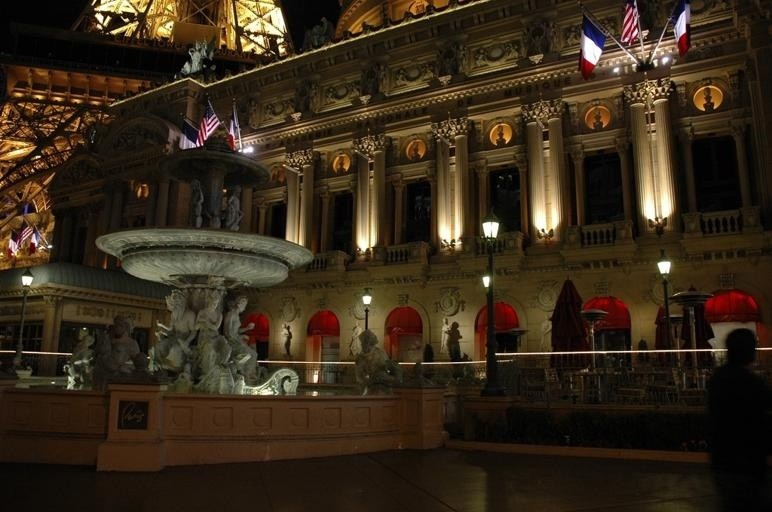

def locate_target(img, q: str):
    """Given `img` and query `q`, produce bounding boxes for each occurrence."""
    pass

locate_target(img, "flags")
[196,102,221,147]
[8,231,20,258]
[619,2,642,45]
[177,119,201,153]
[670,2,695,59]
[576,14,608,83]
[27,226,43,256]
[15,220,33,251]
[227,106,239,150]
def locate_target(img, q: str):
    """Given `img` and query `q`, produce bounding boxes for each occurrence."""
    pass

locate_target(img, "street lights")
[656,248,678,401]
[6,267,34,375]
[479,208,506,396]
[361,287,373,328]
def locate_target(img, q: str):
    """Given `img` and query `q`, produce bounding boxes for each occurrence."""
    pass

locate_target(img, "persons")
[279,323,292,360]
[439,315,451,355]
[61,282,271,393]
[187,176,207,230]
[703,326,772,511]
[225,184,244,230]
[353,329,393,395]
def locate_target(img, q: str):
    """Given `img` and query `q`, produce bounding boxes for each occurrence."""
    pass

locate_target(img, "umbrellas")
[547,275,593,374]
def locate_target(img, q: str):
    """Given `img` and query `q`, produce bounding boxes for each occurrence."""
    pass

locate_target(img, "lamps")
[441,238,455,255]
[537,229,554,244]
[354,247,371,261]
[647,216,667,238]
